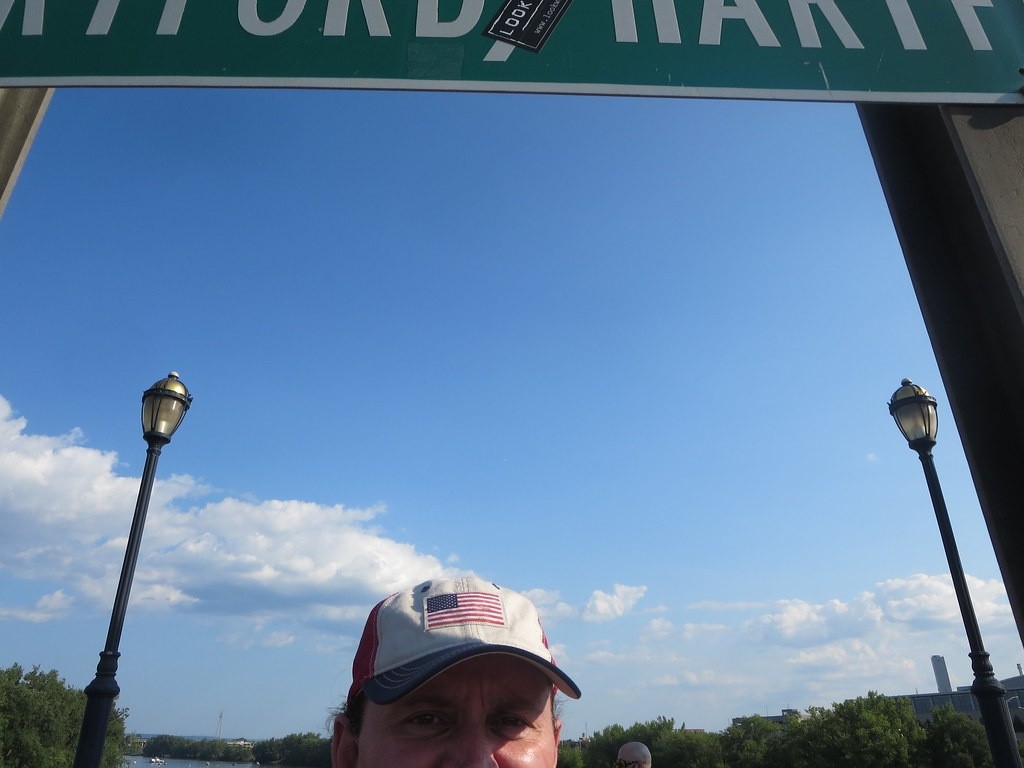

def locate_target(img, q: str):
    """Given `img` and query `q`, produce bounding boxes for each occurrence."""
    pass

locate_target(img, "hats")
[345,576,582,707]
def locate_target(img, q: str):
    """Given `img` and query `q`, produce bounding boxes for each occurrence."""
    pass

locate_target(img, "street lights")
[888,379,1022,768]
[69,369,192,767]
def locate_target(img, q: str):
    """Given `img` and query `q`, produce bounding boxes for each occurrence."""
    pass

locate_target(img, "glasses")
[612,758,647,768]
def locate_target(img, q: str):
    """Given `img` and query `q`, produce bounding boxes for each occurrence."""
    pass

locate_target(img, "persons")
[613,742,652,768]
[330,576,582,768]
